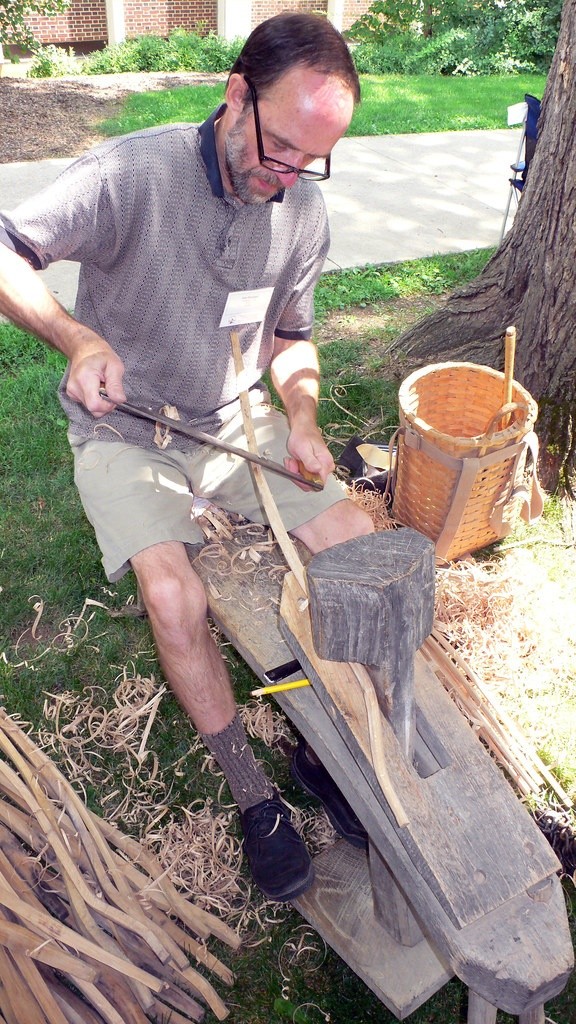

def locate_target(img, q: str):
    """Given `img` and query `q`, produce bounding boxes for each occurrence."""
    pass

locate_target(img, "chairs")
[500,93,541,245]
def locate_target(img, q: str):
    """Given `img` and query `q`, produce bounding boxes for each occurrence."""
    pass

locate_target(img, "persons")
[0,11,375,902]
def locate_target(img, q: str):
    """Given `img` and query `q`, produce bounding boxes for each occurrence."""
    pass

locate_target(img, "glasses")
[243,72,332,182]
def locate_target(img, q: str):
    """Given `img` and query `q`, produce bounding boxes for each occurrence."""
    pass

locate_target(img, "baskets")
[391,359,537,554]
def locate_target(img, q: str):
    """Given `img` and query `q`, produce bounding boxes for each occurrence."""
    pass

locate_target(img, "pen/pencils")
[250,678,311,696]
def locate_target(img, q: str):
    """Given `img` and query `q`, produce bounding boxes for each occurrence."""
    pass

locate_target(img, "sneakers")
[240,786,314,903]
[292,740,368,850]
